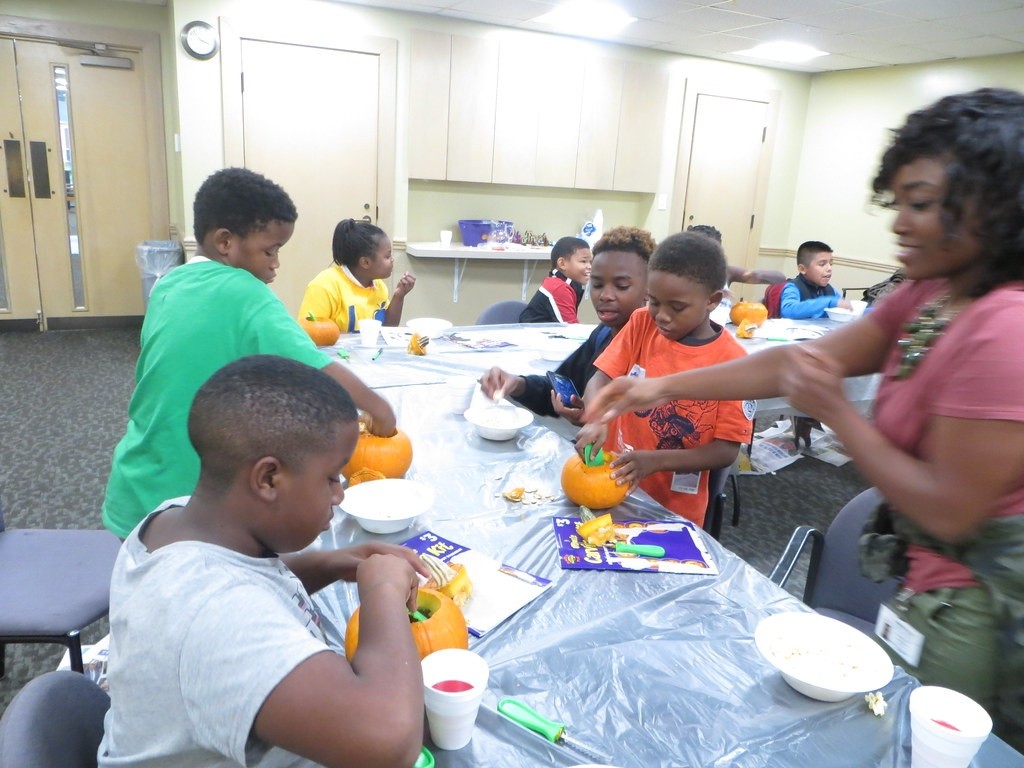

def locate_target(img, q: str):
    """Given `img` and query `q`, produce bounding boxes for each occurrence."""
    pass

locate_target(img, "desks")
[406,242,554,304]
[290,316,1024,768]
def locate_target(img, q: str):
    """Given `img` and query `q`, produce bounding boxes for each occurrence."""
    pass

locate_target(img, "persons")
[688,224,785,323]
[102,166,395,548]
[518,236,591,323]
[480,226,656,444]
[574,231,756,533]
[97,354,431,768]
[299,218,414,333]
[781,241,851,318]
[584,87,1024,755]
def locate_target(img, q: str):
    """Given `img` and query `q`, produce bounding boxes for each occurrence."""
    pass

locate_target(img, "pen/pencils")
[309,312,317,321]
[372,348,384,360]
[767,338,788,341]
[795,338,816,341]
[574,338,588,341]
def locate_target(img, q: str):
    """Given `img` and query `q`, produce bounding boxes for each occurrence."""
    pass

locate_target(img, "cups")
[420,648,489,751]
[358,319,382,345]
[909,685,993,768]
[440,231,452,247]
[709,306,730,328]
[850,300,868,320]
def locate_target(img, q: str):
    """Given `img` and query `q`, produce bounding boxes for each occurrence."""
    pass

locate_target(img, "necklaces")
[890,296,968,380]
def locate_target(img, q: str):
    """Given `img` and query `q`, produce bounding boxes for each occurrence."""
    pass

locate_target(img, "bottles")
[592,208,603,242]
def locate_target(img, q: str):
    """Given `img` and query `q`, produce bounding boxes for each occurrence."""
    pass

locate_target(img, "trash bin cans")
[137,241,184,312]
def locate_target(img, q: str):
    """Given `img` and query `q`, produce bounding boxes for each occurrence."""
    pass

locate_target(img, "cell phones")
[546,371,583,408]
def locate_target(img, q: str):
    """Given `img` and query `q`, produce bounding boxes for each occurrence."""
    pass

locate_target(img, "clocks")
[180,21,220,60]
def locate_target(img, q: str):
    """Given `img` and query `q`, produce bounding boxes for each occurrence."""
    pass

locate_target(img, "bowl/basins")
[753,612,894,703]
[824,307,859,322]
[463,405,534,440]
[406,317,453,339]
[337,479,433,535]
[458,219,514,247]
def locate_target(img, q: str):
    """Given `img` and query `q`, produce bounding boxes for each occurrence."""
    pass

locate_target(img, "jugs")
[488,220,517,251]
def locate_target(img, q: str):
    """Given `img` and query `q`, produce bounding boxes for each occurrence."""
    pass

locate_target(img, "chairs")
[0,506,125,768]
[476,280,903,638]
